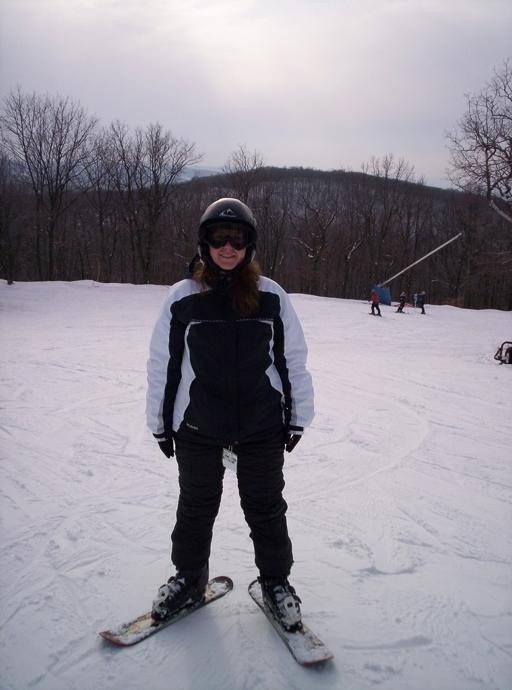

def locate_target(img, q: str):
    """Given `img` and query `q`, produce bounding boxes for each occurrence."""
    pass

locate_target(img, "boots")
[258,576,303,634]
[152,561,208,620]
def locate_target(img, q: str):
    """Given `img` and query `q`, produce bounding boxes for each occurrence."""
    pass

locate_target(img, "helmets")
[199,197,258,235]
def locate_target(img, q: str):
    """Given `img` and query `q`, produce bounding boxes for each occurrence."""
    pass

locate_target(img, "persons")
[397,289,425,314]
[370,289,380,315]
[146,197,314,630]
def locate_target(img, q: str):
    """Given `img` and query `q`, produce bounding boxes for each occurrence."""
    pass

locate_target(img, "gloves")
[158,439,174,458]
[285,435,301,453]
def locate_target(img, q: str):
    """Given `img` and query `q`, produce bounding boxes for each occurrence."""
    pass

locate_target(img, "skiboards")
[100,576,334,664]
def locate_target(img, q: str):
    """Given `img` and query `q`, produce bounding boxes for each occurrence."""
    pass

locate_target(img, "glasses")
[208,225,247,249]
